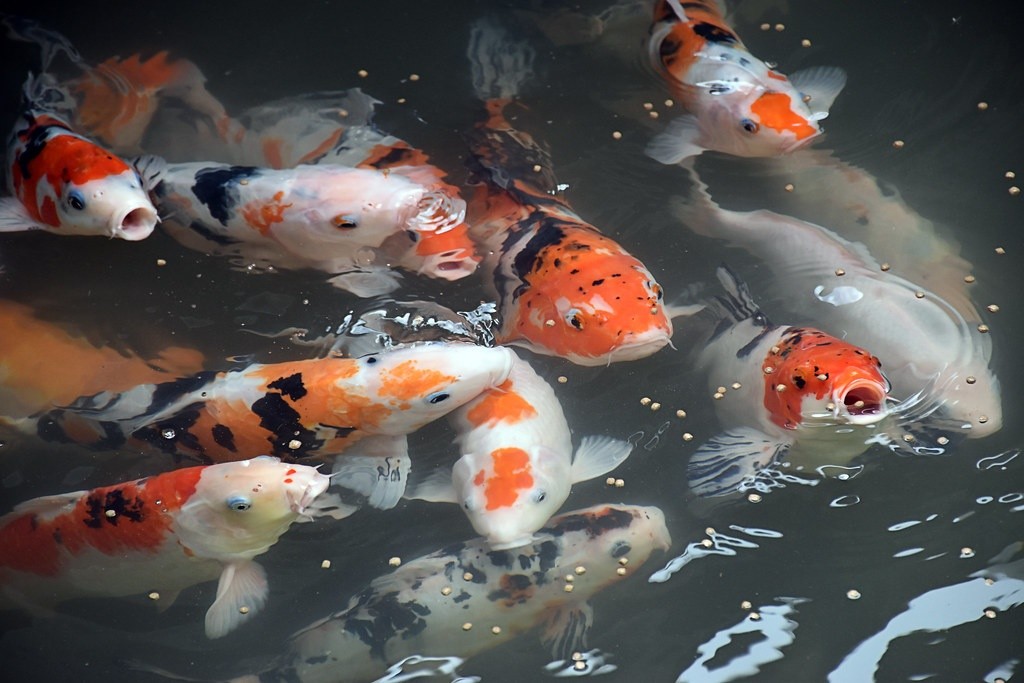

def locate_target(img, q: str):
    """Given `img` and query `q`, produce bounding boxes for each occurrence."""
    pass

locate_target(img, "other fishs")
[0,0,1006,682]
